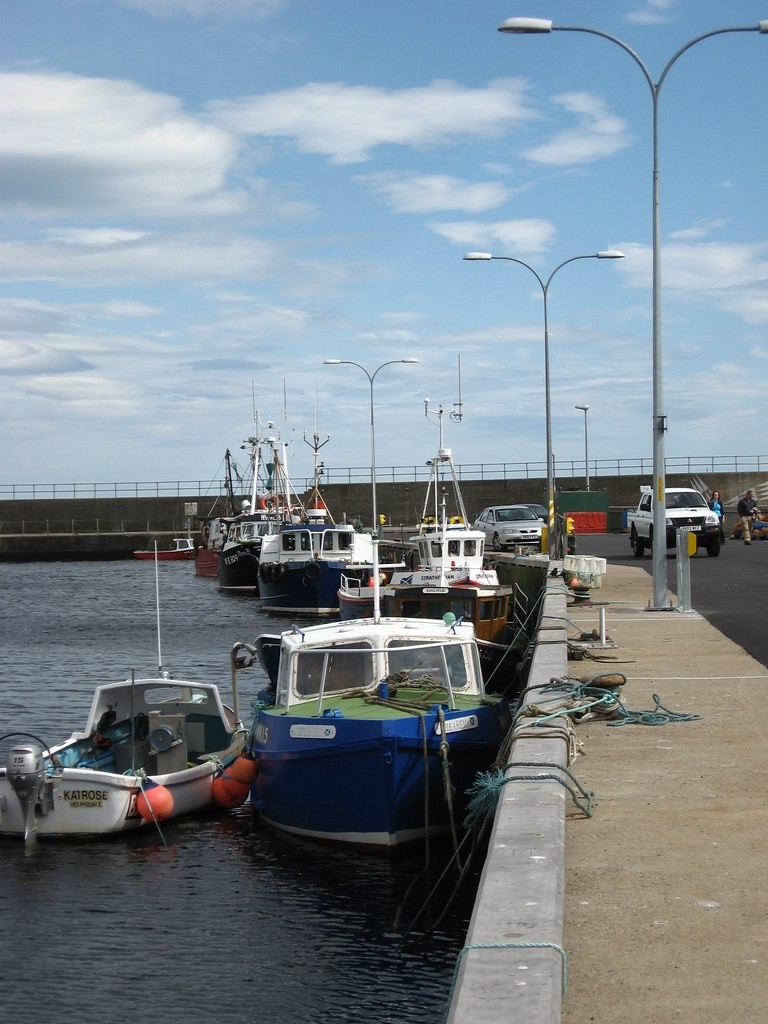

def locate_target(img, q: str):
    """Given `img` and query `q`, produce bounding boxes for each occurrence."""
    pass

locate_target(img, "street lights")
[496,14,767,612]
[574,404,590,492]
[461,250,627,559]
[324,359,424,538]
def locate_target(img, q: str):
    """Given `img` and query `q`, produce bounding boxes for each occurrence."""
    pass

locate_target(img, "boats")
[193,375,514,647]
[228,539,513,853]
[132,518,195,561]
[0,539,248,851]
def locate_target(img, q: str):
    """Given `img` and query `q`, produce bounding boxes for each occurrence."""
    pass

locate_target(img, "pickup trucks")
[626,488,721,558]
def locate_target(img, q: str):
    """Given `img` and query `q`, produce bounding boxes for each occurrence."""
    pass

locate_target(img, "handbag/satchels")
[134,713,149,741]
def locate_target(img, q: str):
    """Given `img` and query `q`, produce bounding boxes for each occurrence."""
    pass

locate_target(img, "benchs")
[77,750,116,772]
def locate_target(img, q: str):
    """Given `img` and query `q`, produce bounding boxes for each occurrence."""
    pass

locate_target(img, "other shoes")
[721,541,725,545]
[745,541,752,545]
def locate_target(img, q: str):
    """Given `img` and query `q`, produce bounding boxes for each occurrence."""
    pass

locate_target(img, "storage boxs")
[563,554,607,573]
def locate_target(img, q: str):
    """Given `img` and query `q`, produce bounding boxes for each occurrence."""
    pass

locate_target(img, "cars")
[509,504,548,525]
[474,505,545,553]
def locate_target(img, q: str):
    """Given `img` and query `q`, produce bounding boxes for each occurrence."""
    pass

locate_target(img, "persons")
[707,491,726,546]
[738,490,757,545]
